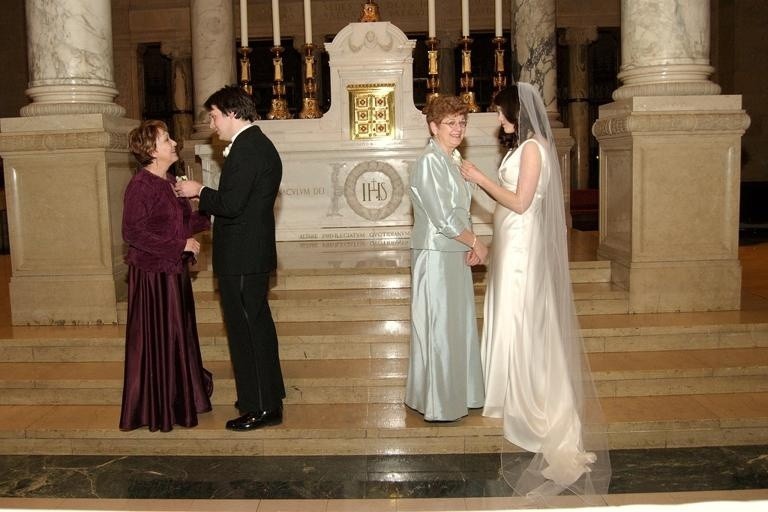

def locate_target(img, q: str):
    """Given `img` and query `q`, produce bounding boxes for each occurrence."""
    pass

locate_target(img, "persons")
[460,82,611,508]
[119,120,213,431]
[174,83,286,431]
[404,95,489,422]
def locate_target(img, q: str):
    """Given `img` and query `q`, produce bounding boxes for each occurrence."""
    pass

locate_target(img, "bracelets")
[470,233,477,248]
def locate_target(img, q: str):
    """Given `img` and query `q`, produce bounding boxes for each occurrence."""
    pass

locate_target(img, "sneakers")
[226,401,283,431]
